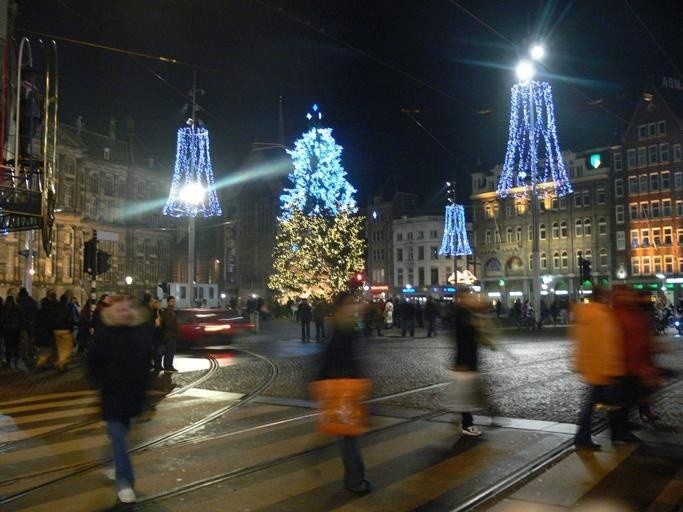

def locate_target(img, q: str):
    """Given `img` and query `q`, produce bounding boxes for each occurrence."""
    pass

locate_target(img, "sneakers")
[118,489,135,503]
[2,354,72,372]
[462,427,481,436]
[106,469,115,480]
[155,367,178,372]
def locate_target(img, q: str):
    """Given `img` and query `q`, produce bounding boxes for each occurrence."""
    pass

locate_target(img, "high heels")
[639,407,658,419]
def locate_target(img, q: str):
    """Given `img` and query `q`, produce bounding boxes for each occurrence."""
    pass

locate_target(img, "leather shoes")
[343,480,370,492]
[611,433,640,442]
[575,439,601,448]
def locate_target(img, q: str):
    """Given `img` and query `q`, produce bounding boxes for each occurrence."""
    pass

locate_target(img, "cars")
[174,308,253,347]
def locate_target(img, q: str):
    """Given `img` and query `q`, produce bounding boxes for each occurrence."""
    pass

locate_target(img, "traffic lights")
[581,258,592,281]
[83,241,113,274]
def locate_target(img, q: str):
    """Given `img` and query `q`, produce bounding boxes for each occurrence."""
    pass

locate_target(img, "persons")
[489,298,561,330]
[313,291,371,492]
[146,289,178,372]
[2,288,109,373]
[200,296,263,318]
[91,302,152,504]
[358,295,454,338]
[573,285,661,449]
[287,295,325,343]
[455,288,497,436]
[653,301,675,335]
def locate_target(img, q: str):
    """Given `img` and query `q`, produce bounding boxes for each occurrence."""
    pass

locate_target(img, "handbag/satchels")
[448,369,481,412]
[311,379,372,436]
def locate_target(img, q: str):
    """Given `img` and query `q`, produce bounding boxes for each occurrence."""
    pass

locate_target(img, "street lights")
[446,177,459,301]
[515,44,545,323]
[183,179,204,308]
